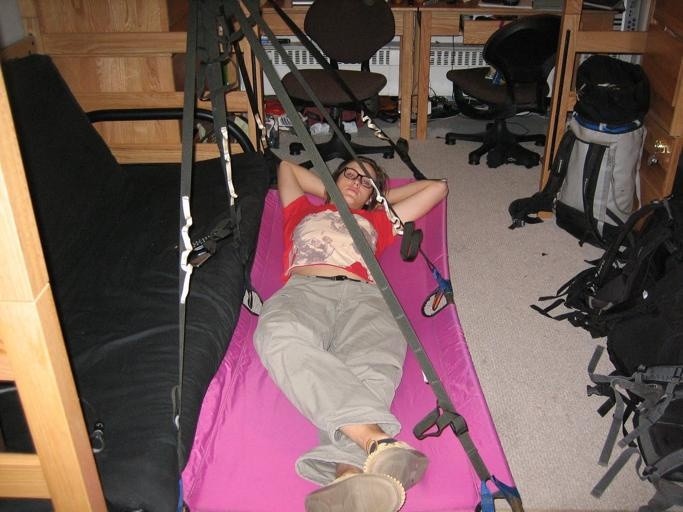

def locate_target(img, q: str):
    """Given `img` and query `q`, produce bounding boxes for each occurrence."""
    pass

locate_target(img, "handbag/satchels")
[573,55,652,127]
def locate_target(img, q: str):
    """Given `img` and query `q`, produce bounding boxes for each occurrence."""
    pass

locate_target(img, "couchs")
[0,50,275,512]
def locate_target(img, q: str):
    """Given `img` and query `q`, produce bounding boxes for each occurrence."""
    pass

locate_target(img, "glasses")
[337,166,374,189]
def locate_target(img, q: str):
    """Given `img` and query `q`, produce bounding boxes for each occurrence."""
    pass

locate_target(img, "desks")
[263,1,419,146]
[239,0,622,139]
[414,2,564,142]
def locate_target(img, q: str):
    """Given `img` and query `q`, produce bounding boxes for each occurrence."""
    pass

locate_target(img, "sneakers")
[362,439,430,493]
[303,471,407,512]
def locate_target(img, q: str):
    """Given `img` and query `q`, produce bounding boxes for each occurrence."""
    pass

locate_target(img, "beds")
[180,178,525,512]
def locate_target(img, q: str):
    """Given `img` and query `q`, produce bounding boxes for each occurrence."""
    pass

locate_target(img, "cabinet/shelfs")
[638,0,683,215]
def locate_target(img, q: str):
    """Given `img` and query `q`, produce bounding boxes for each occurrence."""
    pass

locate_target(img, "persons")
[249,152,447,512]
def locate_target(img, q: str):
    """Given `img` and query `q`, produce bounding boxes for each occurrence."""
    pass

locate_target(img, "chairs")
[276,2,399,170]
[443,10,564,171]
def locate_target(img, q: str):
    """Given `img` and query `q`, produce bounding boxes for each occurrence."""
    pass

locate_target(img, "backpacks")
[562,188,683,512]
[506,109,648,254]
